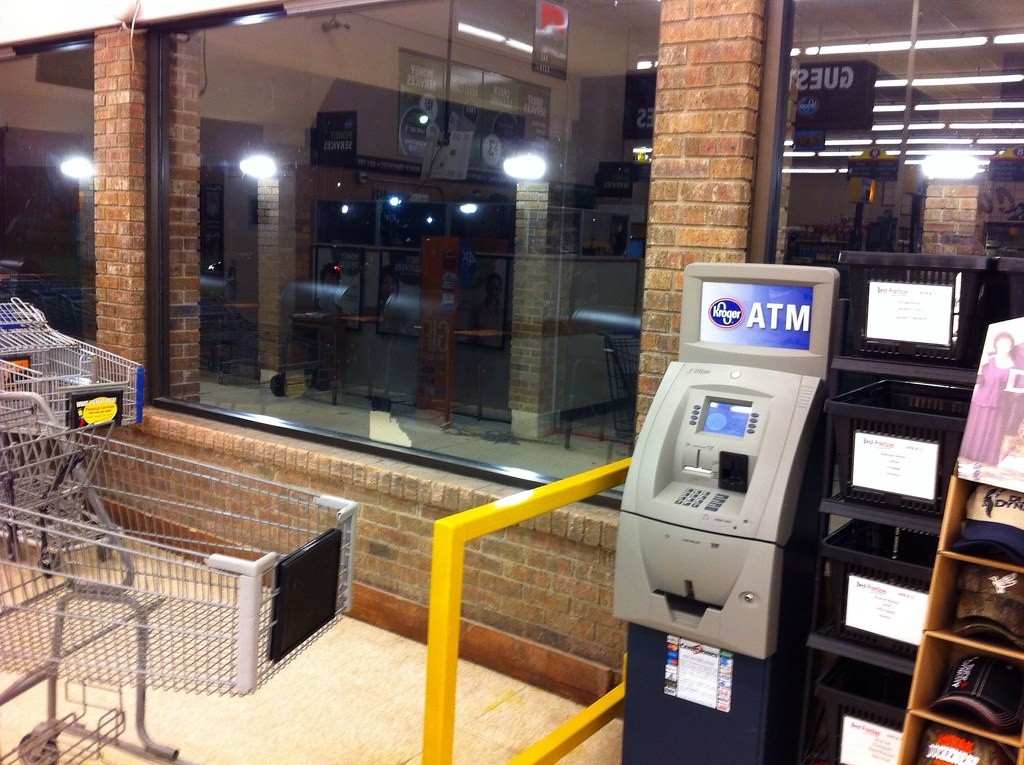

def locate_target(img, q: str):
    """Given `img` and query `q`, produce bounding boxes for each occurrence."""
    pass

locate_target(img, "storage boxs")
[817,518,940,662]
[838,249,1024,368]
[823,379,974,519]
[816,656,913,765]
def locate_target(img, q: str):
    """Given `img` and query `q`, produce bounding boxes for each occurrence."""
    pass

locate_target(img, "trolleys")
[0,296,360,765]
[597,328,641,470]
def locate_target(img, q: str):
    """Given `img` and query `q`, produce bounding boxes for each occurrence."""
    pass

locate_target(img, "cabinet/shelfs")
[799,347,977,765]
[897,476,1024,764]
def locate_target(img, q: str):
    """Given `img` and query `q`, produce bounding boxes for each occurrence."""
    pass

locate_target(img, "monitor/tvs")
[313,198,515,252]
